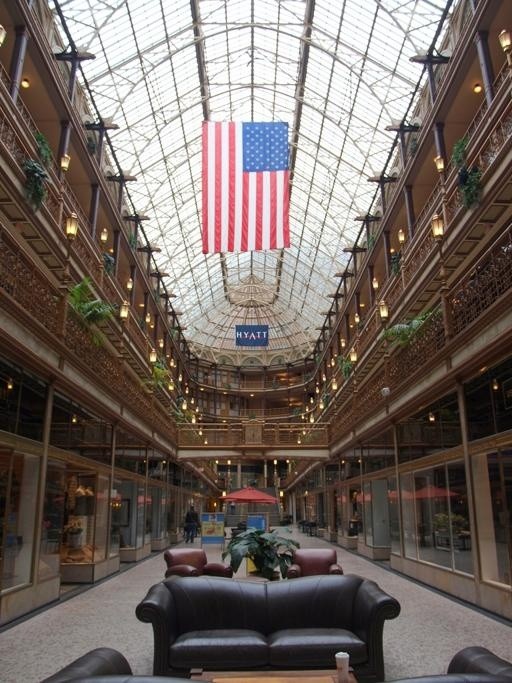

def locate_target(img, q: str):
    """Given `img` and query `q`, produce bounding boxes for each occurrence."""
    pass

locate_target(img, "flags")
[201,122,291,253]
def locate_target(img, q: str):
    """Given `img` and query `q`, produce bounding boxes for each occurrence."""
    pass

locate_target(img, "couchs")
[135,573,400,680]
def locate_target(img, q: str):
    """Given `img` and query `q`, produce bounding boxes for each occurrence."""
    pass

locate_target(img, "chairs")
[164,548,233,578]
[40,647,211,682]
[286,548,343,578]
[384,646,511,682]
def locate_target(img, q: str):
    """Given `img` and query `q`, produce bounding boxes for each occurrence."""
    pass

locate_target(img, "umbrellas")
[219,486,279,511]
[357,485,460,521]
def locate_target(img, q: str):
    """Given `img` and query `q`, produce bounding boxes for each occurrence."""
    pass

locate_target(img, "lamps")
[432,154,446,174]
[19,77,32,92]
[126,277,133,291]
[145,310,150,323]
[331,377,338,388]
[59,154,72,171]
[119,300,131,321]
[429,212,445,242]
[472,84,484,95]
[149,320,155,329]
[330,302,367,368]
[496,26,510,54]
[396,227,406,246]
[64,212,81,242]
[149,348,157,363]
[100,228,109,244]
[376,298,391,323]
[371,277,380,291]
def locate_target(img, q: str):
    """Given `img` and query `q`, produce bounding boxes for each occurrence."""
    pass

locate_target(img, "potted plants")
[222,528,300,580]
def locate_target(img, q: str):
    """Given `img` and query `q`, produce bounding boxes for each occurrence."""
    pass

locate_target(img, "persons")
[184,505,200,544]
[230,502,236,514]
